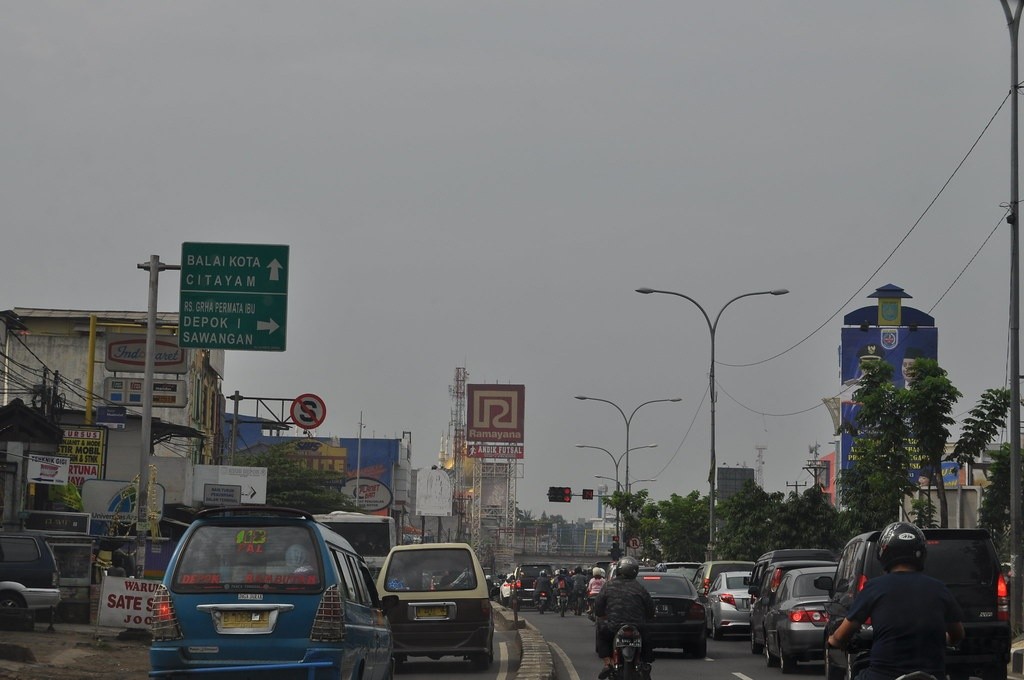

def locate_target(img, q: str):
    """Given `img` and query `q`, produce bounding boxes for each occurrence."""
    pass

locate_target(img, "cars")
[697,571,758,641]
[760,567,838,674]
[749,559,839,657]
[499,573,516,604]
[633,572,708,659]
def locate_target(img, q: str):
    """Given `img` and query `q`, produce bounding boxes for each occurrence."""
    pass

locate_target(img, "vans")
[509,563,553,612]
[375,542,495,671]
[692,560,756,597]
[748,548,836,604]
[814,527,1012,680]
[147,505,395,679]
[587,561,703,584]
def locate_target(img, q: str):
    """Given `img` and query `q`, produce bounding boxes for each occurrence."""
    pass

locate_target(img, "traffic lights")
[546,486,572,502]
[582,488,593,499]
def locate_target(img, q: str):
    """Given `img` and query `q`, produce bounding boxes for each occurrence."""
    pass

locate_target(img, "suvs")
[0,533,61,631]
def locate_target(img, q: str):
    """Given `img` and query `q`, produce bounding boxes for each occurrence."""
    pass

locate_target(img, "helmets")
[486,575,491,580]
[592,567,601,576]
[654,563,667,572]
[600,568,605,579]
[539,567,592,577]
[876,521,927,571]
[616,555,639,578]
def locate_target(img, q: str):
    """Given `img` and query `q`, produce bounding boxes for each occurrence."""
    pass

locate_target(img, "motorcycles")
[586,609,652,680]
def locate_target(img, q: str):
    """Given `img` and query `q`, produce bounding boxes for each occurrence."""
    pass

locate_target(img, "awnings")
[401,522,422,534]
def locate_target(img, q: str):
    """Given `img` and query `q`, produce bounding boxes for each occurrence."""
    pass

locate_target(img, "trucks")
[301,511,399,585]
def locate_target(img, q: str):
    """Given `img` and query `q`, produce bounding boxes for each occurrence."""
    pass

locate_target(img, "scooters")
[821,602,961,680]
[538,589,606,622]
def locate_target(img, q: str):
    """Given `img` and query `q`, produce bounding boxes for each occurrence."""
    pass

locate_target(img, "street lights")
[574,395,683,495]
[592,475,658,549]
[575,443,657,549]
[633,286,791,564]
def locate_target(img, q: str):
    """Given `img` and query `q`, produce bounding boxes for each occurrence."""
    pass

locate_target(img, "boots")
[598,657,613,680]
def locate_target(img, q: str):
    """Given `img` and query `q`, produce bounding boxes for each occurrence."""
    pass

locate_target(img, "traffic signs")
[177,242,290,351]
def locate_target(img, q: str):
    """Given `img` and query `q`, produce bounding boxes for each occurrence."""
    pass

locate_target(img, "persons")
[595,556,655,680]
[827,521,964,680]
[485,574,500,603]
[349,526,387,557]
[532,568,574,611]
[582,566,608,613]
[569,564,589,615]
